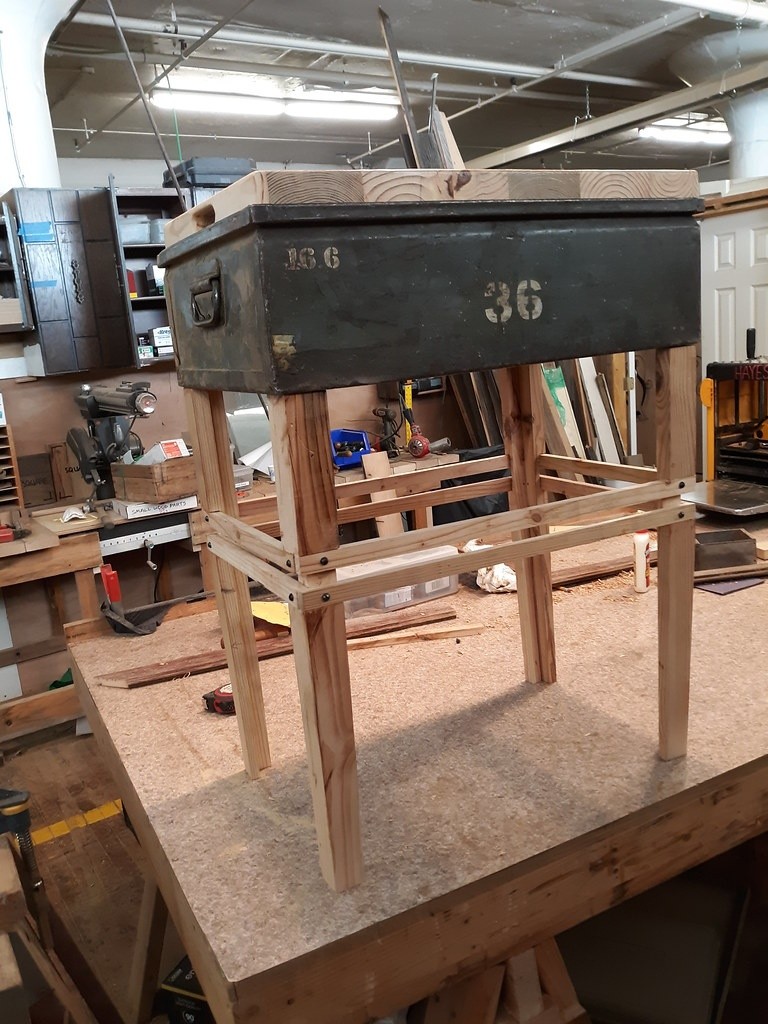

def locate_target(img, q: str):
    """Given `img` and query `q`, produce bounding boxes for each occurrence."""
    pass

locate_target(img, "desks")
[1,452,460,744]
[155,168,703,893]
[62,510,768,1024]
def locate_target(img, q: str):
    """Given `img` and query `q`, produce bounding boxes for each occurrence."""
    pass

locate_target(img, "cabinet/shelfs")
[0,173,233,378]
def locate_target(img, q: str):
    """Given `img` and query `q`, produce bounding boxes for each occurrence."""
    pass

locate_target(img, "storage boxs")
[334,545,460,615]
[162,157,257,188]
[110,455,198,504]
[231,464,255,493]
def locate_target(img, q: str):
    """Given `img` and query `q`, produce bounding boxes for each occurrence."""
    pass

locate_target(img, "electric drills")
[369,407,399,459]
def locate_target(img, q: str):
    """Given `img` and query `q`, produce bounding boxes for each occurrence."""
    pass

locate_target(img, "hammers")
[82,499,116,531]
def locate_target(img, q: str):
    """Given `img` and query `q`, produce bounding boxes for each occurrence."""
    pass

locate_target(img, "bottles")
[632,529,650,594]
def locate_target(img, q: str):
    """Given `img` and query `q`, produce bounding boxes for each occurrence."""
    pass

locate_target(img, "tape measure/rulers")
[202,681,236,715]
